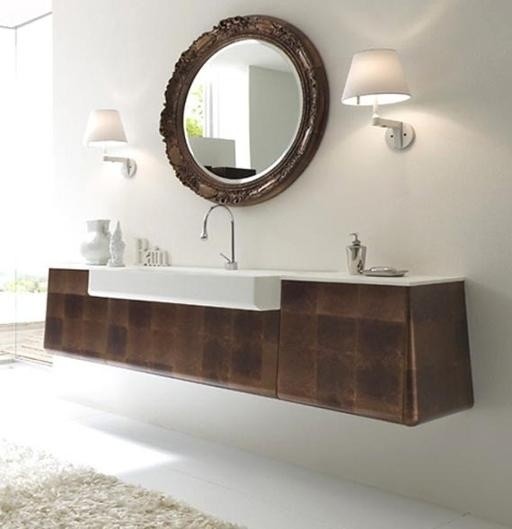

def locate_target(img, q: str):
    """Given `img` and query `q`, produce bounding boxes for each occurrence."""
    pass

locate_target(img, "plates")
[359,270,410,277]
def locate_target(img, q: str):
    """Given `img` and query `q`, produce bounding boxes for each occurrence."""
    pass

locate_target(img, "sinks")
[89,267,281,311]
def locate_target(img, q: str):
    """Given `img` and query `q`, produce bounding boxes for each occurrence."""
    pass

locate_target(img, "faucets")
[201,203,238,271]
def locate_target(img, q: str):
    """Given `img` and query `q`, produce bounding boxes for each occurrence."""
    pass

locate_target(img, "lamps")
[86,109,138,178]
[342,50,416,151]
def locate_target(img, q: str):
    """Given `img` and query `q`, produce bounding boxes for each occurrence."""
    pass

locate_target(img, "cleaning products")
[344,231,367,276]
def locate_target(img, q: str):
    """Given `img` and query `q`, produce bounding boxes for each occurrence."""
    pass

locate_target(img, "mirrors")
[161,13,329,211]
